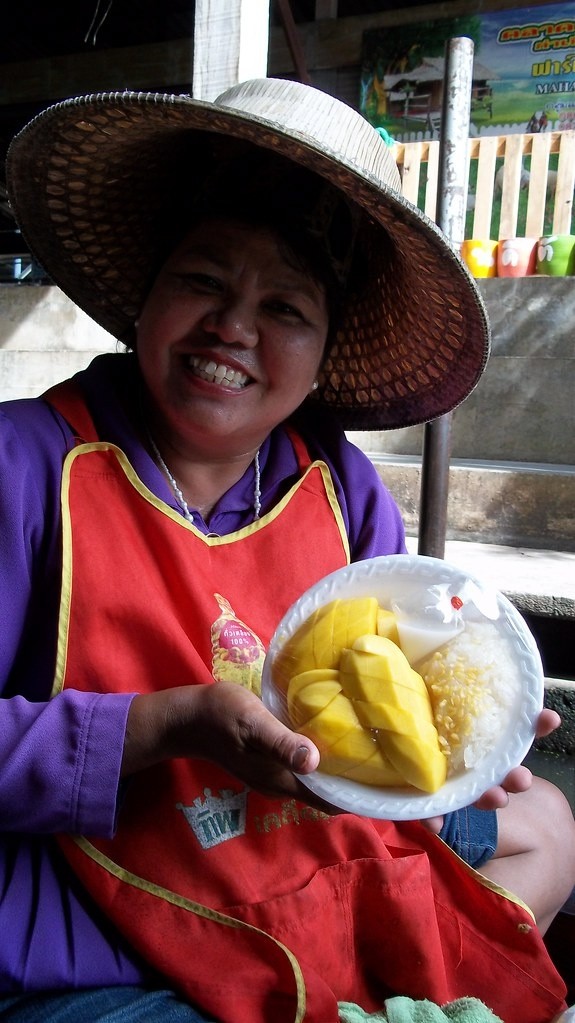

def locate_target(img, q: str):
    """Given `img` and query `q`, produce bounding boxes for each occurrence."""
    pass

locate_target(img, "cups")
[461,240,499,278]
[497,237,537,277]
[535,234,575,276]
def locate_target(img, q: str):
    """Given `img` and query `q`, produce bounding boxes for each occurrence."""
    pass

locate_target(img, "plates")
[261,554,545,821]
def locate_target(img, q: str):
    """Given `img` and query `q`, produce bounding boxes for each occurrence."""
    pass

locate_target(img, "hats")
[2,76,494,432]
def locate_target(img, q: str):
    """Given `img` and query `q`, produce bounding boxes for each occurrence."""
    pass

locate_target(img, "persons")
[0,125,573,1023]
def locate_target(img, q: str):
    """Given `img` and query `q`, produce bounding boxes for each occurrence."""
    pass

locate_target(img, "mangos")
[272,596,447,793]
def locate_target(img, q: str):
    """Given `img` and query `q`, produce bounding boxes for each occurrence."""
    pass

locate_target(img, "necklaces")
[132,392,262,541]
